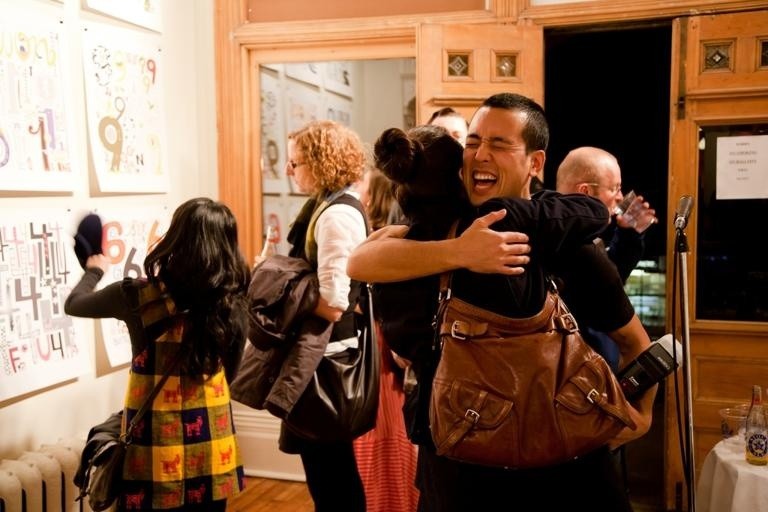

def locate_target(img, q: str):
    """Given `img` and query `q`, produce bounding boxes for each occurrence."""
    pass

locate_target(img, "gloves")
[73,214,102,272]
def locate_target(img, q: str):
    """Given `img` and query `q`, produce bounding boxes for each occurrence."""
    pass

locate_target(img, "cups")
[717,404,751,452]
[610,190,654,233]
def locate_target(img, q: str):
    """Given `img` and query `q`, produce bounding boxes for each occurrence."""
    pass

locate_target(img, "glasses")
[577,182,622,195]
[290,159,307,168]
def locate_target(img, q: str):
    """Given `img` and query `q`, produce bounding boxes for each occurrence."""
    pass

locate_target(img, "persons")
[347,93,663,512]
[64,197,252,512]
[253,119,371,512]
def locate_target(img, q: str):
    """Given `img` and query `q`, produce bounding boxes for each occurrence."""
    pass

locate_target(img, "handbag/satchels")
[283,288,377,449]
[426,220,637,471]
[72,411,132,511]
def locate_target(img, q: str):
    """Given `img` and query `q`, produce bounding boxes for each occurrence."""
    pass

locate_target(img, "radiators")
[0,435,116,512]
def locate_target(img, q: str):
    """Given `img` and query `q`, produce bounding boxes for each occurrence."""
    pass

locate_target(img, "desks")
[709,430,767,512]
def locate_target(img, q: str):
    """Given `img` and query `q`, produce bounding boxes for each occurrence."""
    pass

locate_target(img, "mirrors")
[239,38,416,257]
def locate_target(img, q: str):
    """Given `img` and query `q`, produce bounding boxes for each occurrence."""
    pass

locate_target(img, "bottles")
[260,226,278,258]
[746,385,768,465]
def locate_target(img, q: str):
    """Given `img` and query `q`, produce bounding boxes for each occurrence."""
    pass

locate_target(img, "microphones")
[673,188,695,235]
